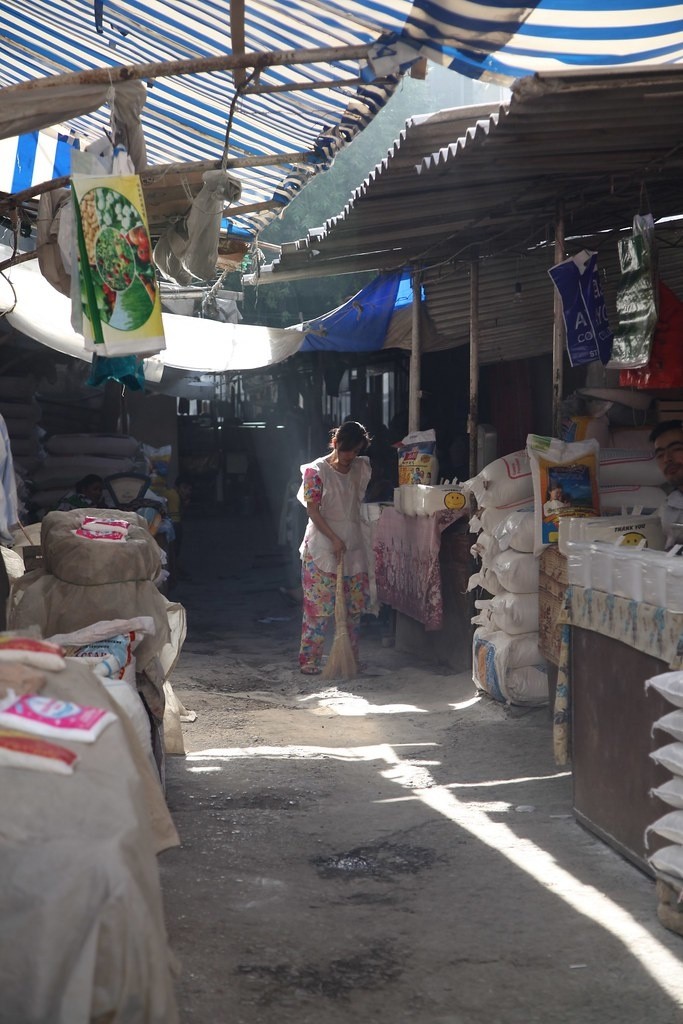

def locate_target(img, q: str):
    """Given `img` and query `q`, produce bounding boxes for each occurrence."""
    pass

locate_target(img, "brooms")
[318,550,358,681]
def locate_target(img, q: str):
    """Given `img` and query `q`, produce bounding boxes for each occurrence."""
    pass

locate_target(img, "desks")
[369,506,494,673]
[553,584,683,880]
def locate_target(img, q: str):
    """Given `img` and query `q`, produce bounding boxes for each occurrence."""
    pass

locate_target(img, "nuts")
[76,190,103,264]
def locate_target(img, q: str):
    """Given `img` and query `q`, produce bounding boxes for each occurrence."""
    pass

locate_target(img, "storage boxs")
[394,483,470,517]
[558,513,666,558]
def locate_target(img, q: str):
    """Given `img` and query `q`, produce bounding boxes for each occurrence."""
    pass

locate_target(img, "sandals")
[355,660,367,674]
[301,662,320,675]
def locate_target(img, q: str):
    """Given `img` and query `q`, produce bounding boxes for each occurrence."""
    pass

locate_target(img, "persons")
[543,483,564,516]
[298,422,372,674]
[0,413,18,632]
[77,474,105,505]
[647,420,683,543]
[413,468,431,485]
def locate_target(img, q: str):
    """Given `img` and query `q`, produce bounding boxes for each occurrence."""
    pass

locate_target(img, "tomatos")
[126,226,150,263]
